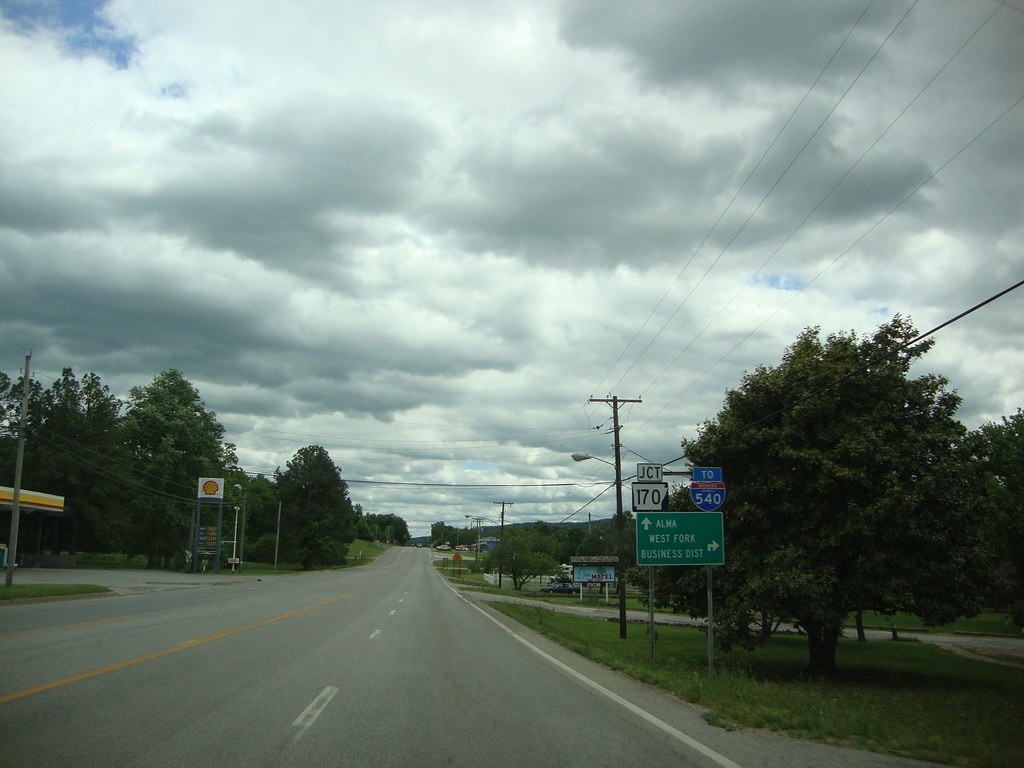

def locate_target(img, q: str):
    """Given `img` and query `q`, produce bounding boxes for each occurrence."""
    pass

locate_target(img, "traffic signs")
[636,511,725,566]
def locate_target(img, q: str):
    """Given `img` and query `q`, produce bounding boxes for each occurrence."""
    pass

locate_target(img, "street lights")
[464,514,503,589]
[570,453,627,640]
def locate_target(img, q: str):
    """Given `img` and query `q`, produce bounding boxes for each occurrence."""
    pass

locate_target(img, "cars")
[541,583,581,594]
[436,543,489,552]
